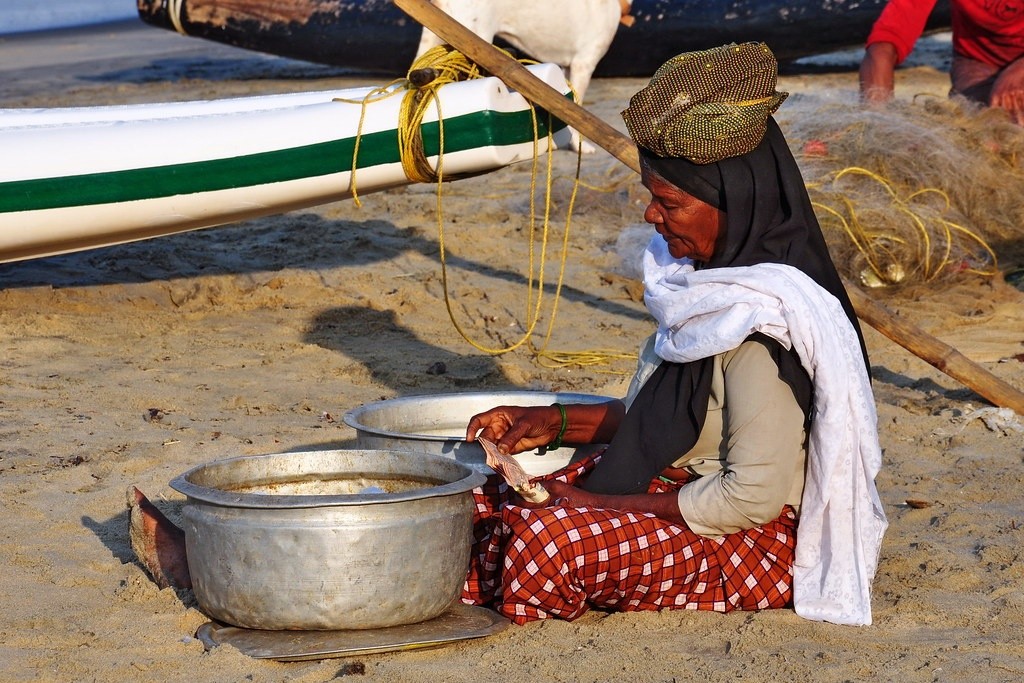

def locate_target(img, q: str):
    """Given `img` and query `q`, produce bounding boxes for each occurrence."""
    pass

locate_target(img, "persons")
[859,0,1024,128]
[457,42,889,626]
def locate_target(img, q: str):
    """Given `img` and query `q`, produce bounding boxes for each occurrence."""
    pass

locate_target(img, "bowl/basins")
[343,391,619,479]
[166,451,486,630]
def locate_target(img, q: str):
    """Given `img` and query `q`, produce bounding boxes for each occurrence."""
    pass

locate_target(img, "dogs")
[405,0,634,152]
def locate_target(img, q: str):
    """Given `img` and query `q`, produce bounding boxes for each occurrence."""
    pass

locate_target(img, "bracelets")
[547,403,567,451]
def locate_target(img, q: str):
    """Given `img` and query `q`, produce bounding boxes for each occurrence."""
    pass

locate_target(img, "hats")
[620,42,790,164]
